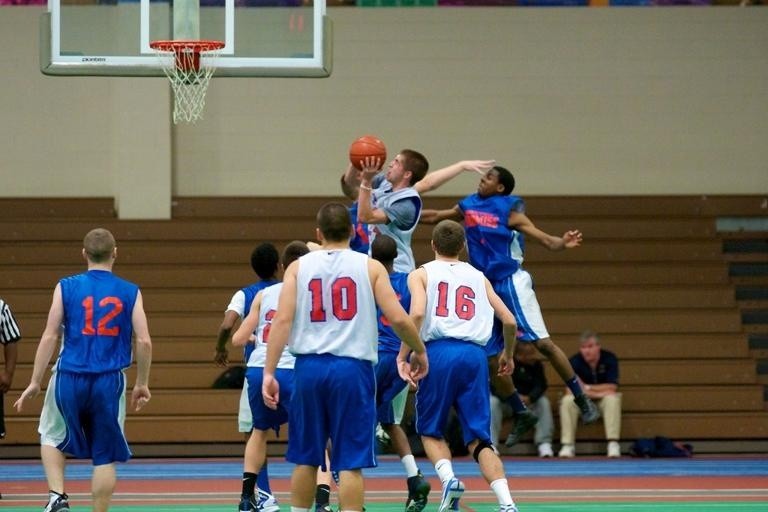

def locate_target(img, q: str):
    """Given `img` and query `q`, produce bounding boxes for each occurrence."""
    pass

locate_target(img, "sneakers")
[375,422,390,445]
[573,393,601,425]
[44,492,71,511]
[239,499,257,511]
[559,444,575,458]
[438,476,465,512]
[256,498,280,511]
[505,407,539,447]
[607,441,621,458]
[499,503,518,511]
[405,475,431,512]
[537,443,554,458]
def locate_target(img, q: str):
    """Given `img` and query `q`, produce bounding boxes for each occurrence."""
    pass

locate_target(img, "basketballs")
[349,134,386,171]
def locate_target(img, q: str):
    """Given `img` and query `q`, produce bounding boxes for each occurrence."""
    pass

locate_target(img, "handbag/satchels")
[210,366,245,388]
[629,436,694,458]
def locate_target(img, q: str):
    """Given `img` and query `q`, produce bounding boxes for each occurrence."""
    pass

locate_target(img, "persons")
[416,166,602,427]
[556,330,624,461]
[12,227,153,512]
[215,204,429,512]
[341,148,498,443]
[485,337,556,460]
[0,296,23,502]
